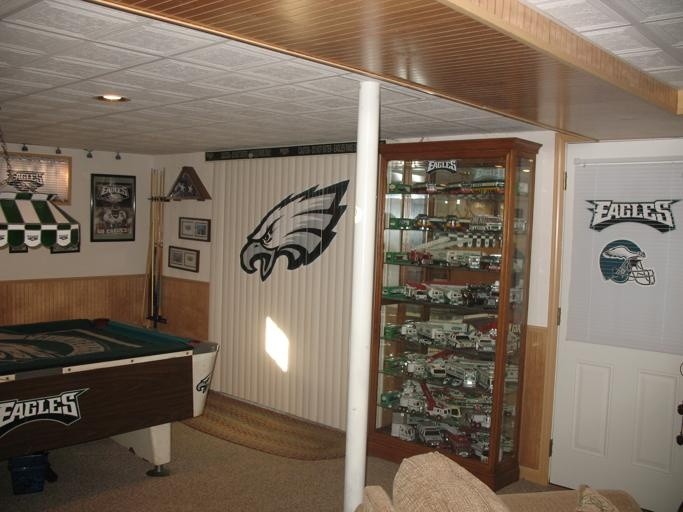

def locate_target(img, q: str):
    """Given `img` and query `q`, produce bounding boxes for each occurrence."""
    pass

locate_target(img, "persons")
[101,203,129,227]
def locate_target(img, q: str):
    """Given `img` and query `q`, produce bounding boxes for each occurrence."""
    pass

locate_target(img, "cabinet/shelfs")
[367,139,538,490]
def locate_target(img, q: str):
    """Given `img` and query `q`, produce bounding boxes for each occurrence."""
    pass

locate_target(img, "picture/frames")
[89,172,136,244]
[168,217,211,273]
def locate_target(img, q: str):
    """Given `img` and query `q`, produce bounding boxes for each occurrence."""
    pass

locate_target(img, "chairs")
[363,450,641,511]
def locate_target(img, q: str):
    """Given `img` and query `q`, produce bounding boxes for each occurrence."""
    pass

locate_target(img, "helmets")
[599,239,646,286]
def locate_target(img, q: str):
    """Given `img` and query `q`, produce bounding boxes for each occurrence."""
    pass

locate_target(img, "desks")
[0,314,219,494]
